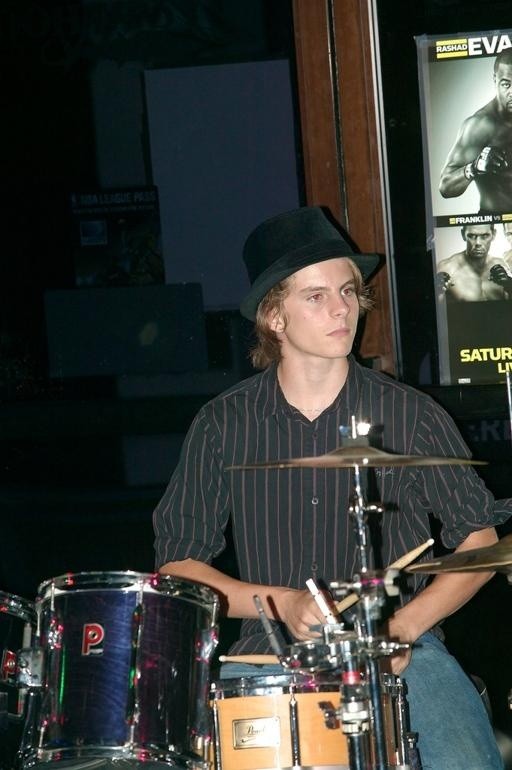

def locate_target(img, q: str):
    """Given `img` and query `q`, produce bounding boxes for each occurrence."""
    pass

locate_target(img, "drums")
[205,676,410,770]
[21,572,219,769]
[2,592,35,725]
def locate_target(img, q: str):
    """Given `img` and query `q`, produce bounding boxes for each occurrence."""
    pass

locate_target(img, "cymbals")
[406,540,511,574]
[226,444,486,468]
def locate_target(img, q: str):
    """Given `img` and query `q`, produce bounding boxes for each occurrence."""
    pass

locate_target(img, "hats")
[233,202,389,325]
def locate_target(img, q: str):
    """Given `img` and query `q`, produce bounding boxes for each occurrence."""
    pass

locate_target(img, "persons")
[431,222,511,323]
[437,49,512,215]
[148,206,511,770]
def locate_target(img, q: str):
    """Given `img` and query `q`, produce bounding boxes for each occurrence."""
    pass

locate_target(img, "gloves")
[436,269,451,297]
[488,262,512,293]
[463,144,510,182]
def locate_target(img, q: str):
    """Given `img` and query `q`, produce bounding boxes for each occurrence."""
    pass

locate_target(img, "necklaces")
[297,408,323,415]
